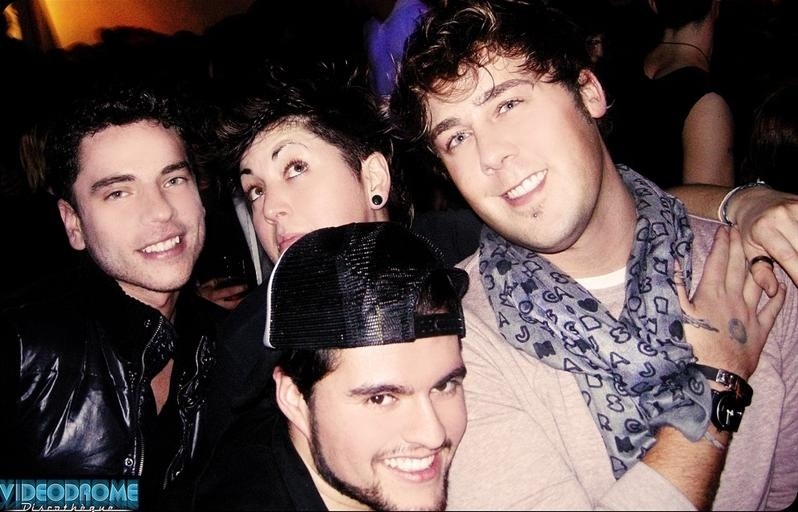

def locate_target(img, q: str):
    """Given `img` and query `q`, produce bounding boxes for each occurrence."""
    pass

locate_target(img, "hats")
[206,222,469,407]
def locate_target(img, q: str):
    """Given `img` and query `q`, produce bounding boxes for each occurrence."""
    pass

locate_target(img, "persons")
[0,0,798,512]
